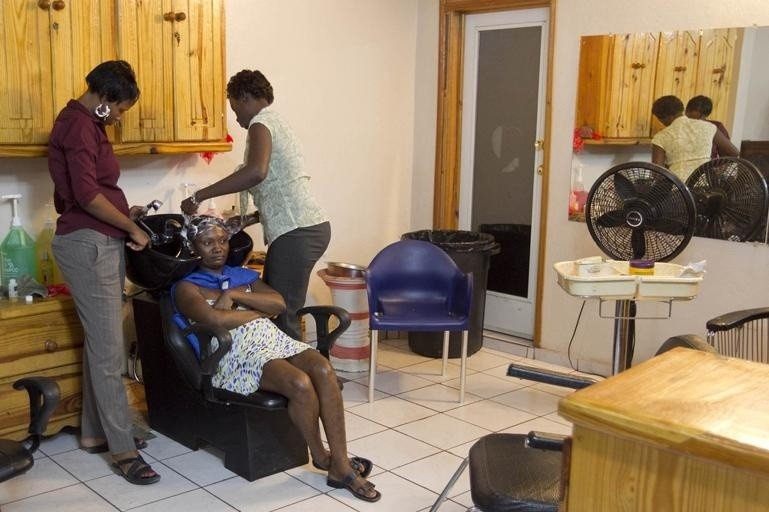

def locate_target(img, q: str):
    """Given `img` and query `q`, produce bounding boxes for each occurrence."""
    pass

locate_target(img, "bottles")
[8,278,19,298]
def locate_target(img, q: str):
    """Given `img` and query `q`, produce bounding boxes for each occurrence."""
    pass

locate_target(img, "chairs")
[158,285,352,482]
[364,239,474,404]
[429,333,721,511]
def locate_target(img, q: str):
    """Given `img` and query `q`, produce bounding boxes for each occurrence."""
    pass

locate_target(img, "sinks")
[124,213,252,288]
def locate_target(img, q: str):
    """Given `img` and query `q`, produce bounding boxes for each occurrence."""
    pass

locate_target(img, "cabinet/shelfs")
[0,283,150,443]
[573,28,737,147]
[0,1,233,159]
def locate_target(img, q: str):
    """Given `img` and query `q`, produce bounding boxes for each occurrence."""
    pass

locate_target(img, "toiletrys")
[0,194,35,289]
[35,202,67,286]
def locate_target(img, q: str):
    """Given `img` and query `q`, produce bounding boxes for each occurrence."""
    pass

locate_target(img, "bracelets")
[190,191,199,206]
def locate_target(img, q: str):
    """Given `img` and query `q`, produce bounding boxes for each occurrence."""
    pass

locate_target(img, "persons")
[649,94,740,190]
[176,66,332,344]
[167,212,383,503]
[684,95,731,179]
[44,59,161,486]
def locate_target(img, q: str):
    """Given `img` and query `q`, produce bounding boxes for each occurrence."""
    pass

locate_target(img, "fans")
[676,156,768,242]
[584,160,697,263]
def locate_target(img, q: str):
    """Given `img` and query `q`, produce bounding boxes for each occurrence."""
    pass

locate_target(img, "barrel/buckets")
[316,267,372,373]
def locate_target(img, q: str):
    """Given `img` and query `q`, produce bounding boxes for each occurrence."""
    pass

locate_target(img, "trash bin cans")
[401,230,500,359]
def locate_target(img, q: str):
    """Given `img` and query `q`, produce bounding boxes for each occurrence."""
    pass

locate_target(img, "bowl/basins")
[323,261,366,278]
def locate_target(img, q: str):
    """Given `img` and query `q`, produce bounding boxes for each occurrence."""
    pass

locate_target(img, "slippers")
[313,456,373,478]
[112,455,161,485]
[327,471,381,503]
[86,437,148,454]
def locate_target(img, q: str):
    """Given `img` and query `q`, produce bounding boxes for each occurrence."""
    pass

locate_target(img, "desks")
[557,344,769,512]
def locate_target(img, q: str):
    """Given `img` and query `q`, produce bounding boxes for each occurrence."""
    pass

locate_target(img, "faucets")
[160,217,181,232]
[181,180,196,188]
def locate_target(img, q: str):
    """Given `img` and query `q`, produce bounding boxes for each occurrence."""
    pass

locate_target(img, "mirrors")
[568,25,769,244]
